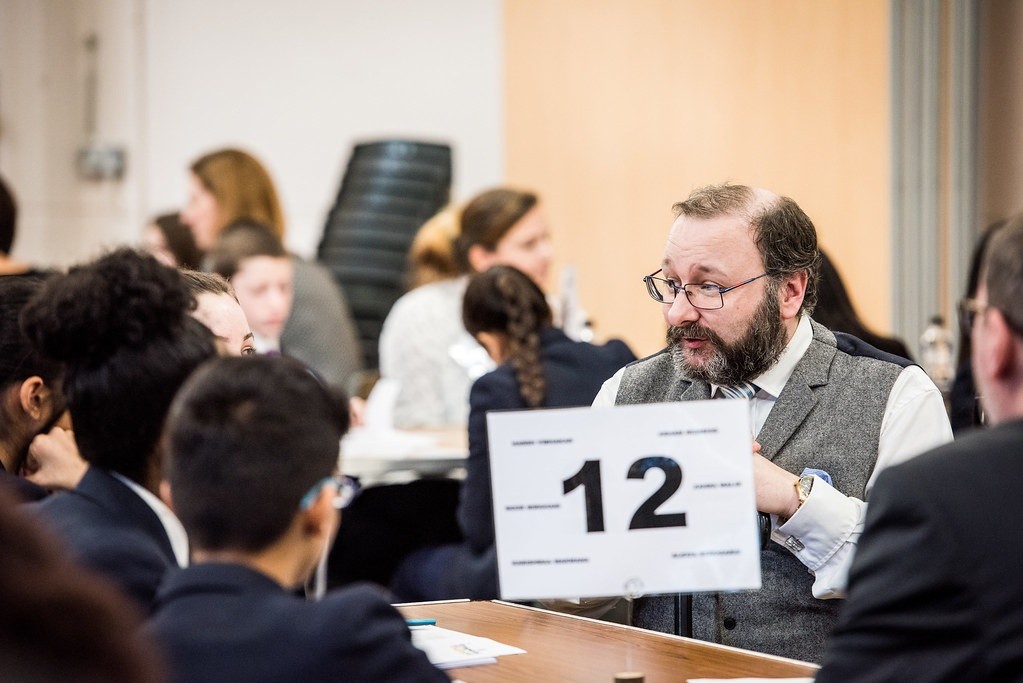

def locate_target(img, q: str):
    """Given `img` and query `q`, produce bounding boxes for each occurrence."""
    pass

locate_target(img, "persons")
[134,351,454,683]
[587,186,954,668]
[1,169,257,683]
[321,188,590,591]
[146,143,472,426]
[812,212,1022,683]
[394,266,637,609]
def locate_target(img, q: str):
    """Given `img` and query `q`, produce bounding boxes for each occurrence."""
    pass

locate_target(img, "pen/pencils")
[406,618,436,626]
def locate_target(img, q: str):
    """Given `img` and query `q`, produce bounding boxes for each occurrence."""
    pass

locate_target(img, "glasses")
[299,473,363,513]
[642,268,775,310]
[961,298,1013,335]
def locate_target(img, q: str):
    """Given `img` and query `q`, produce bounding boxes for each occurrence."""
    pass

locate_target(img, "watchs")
[776,475,815,527]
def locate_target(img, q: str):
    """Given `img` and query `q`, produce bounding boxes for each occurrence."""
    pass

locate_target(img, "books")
[407,622,497,672]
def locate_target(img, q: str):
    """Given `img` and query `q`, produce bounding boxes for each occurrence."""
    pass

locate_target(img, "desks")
[385,591,823,683]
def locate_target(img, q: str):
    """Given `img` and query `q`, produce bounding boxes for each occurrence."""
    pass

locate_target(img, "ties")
[713,380,761,403]
